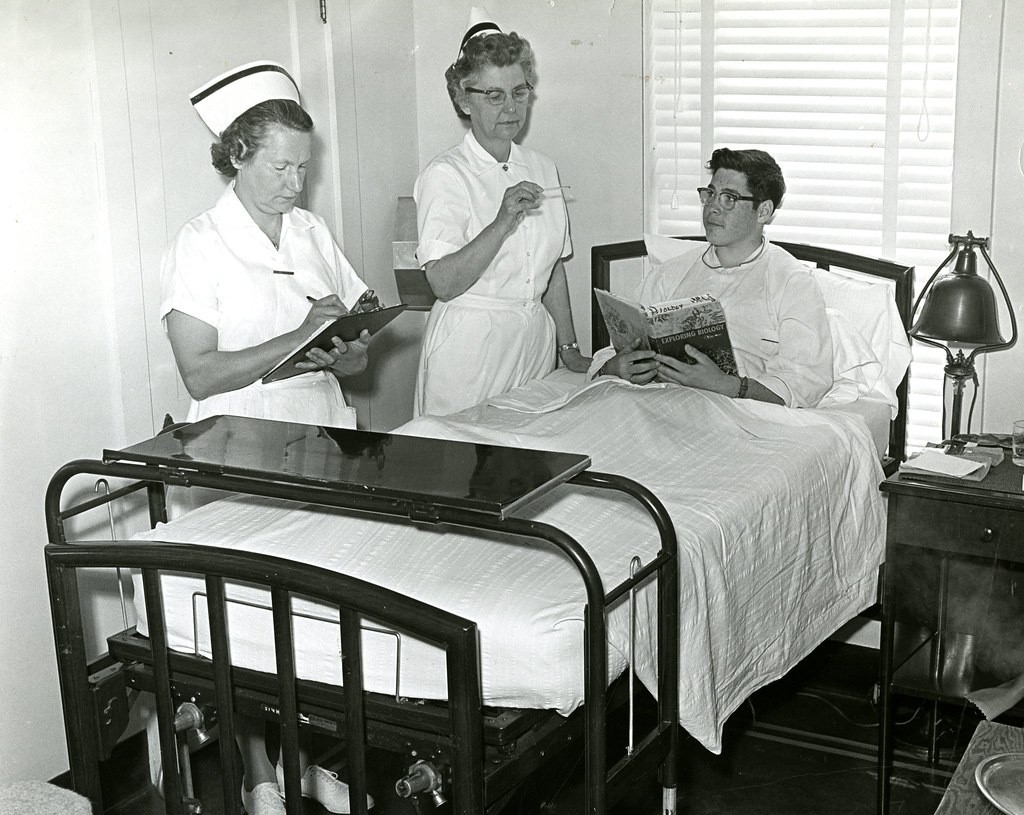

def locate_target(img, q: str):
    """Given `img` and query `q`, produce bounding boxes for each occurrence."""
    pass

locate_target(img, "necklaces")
[701,236,766,269]
[255,216,281,250]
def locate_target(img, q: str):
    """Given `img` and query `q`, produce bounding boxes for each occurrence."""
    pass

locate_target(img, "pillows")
[635,231,915,419]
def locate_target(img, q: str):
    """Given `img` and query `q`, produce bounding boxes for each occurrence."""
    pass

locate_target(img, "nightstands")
[878,441,1024,815]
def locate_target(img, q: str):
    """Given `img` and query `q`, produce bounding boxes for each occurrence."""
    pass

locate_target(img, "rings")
[328,358,337,368]
[629,373,636,383]
[339,342,350,354]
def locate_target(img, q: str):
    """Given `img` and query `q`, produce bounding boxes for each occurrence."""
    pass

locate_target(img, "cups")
[1011,420,1024,467]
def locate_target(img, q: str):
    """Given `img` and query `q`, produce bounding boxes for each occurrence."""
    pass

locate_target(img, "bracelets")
[598,359,607,378]
[734,375,749,398]
[557,341,580,354]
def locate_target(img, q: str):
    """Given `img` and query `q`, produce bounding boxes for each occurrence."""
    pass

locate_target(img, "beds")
[44,235,914,815]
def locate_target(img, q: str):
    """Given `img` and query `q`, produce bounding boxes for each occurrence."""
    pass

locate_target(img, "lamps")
[908,228,1017,447]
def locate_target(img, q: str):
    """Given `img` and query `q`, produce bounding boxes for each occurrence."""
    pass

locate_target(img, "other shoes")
[241,762,375,814]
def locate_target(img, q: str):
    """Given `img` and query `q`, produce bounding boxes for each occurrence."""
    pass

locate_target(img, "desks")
[932,720,1024,815]
[99,416,592,522]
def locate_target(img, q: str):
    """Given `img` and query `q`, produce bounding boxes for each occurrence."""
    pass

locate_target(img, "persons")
[468,444,564,504]
[587,149,835,409]
[159,60,384,815]
[412,7,595,422]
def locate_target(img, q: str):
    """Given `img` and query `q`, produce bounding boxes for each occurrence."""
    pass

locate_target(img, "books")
[898,444,1006,483]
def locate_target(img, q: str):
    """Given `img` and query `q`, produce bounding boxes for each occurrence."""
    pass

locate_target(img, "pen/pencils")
[305,296,317,304]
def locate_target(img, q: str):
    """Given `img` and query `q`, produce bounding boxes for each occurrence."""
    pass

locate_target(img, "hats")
[187,59,304,139]
[454,5,505,63]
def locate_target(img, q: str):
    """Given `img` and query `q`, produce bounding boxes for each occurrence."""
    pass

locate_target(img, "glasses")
[465,81,534,106]
[697,185,770,210]
